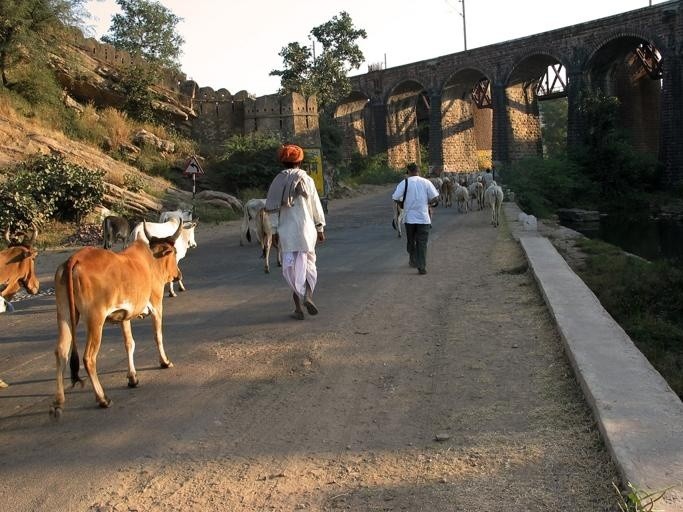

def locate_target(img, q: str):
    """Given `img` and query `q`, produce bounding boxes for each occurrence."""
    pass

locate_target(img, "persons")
[265,144,326,320]
[391,162,439,274]
[481,168,493,185]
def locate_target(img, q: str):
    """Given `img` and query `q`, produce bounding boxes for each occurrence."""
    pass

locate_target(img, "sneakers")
[291,311,305,320]
[418,267,427,274]
[305,299,318,316]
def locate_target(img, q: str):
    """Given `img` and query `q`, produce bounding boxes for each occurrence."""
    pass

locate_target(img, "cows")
[392,199,405,237]
[427,172,504,228]
[0,222,40,388]
[239,194,331,273]
[48,205,201,422]
